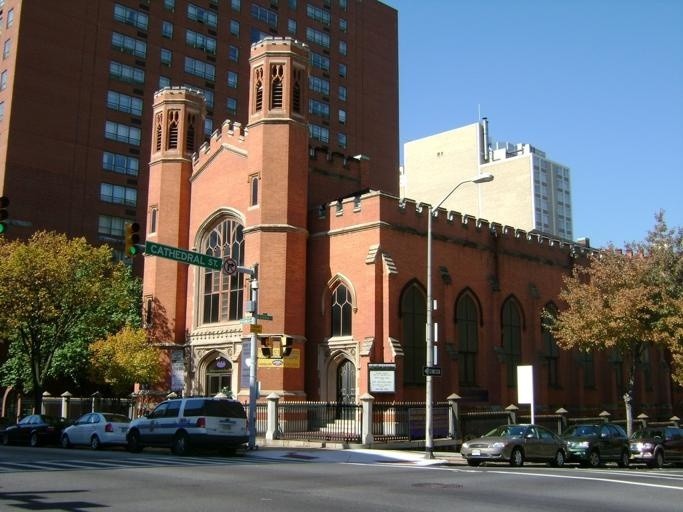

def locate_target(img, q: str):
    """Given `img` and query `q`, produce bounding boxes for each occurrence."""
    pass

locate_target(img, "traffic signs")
[145,241,222,271]
[256,314,273,320]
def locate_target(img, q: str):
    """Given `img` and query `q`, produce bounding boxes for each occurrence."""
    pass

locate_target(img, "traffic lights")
[260,336,272,358]
[0,196,9,236]
[123,221,140,258]
[279,337,292,357]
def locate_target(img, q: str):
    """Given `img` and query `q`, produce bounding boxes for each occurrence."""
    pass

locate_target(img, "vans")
[125,396,249,455]
[629,426,682,467]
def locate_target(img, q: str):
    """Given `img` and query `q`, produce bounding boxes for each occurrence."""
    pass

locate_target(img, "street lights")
[424,175,493,456]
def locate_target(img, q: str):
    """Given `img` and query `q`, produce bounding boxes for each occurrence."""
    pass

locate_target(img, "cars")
[460,423,568,467]
[0,415,65,446]
[58,411,130,449]
[558,422,631,468]
